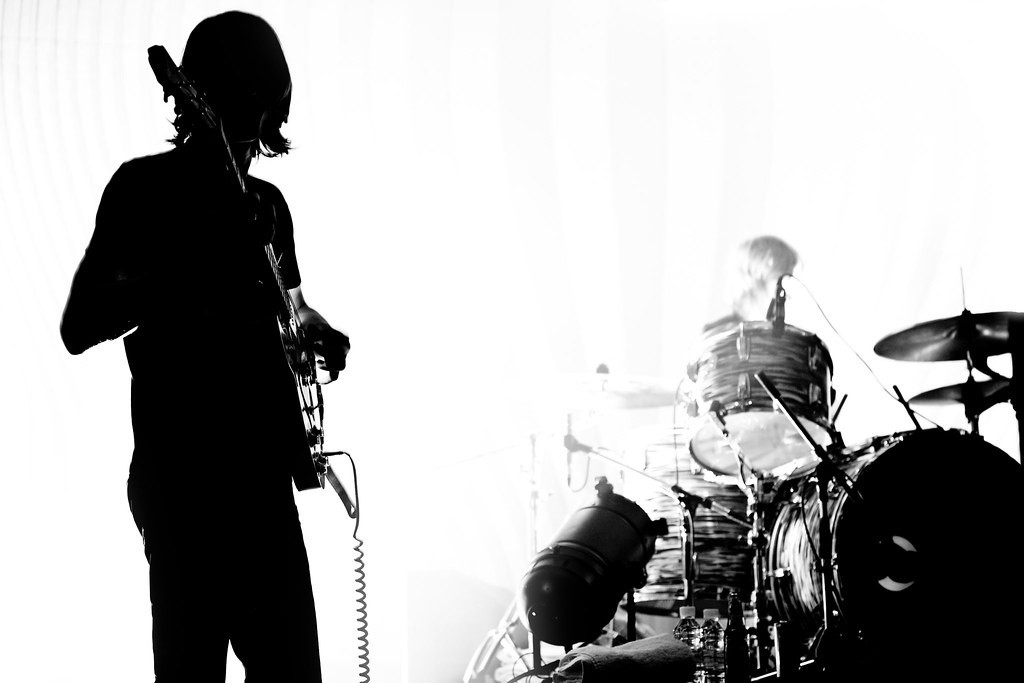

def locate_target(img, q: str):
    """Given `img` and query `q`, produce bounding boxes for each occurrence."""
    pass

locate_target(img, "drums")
[745,424,1024,683]
[687,318,835,482]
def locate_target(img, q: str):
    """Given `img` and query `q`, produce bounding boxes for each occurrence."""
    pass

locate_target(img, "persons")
[60,13,351,683]
[731,230,797,323]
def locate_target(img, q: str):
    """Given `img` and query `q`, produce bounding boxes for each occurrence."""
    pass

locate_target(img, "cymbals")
[872,310,1024,363]
[905,377,1015,406]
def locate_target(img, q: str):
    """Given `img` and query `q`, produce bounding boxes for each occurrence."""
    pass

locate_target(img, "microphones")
[564,436,579,454]
[771,275,786,338]
[877,536,923,585]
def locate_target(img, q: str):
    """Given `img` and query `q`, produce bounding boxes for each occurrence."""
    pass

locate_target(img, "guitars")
[146,44,331,492]
